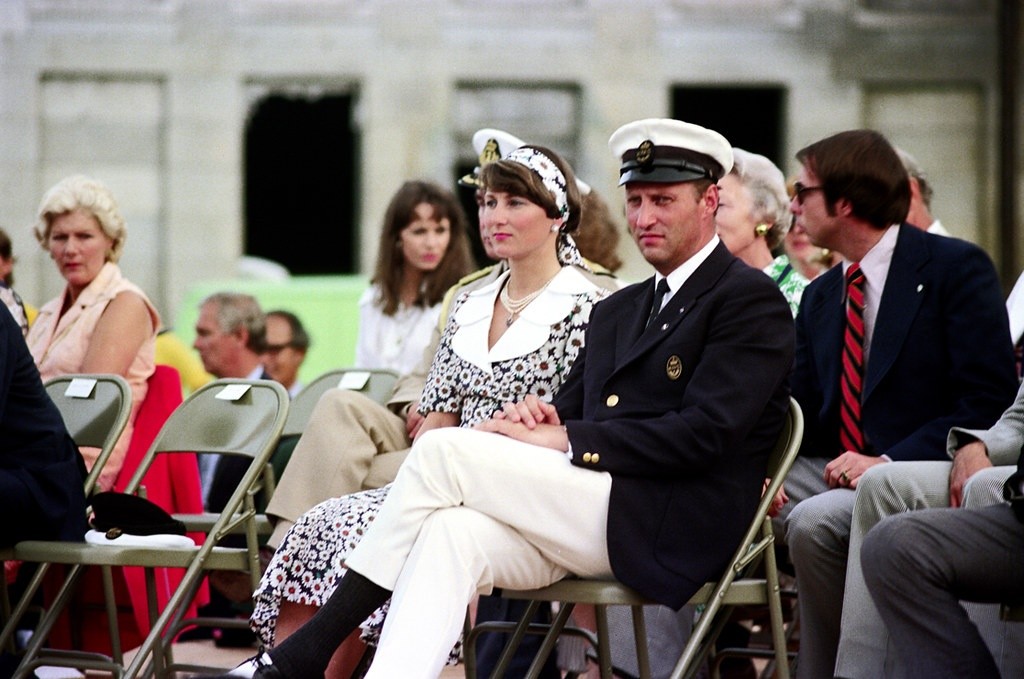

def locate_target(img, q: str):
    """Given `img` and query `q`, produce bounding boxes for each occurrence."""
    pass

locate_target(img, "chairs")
[0,367,804,679]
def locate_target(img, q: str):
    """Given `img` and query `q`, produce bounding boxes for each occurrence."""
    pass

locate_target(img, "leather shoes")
[191,648,281,679]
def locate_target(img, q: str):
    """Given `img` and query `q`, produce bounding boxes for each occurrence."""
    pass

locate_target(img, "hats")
[609,117,737,188]
[458,129,589,204]
[84,491,195,548]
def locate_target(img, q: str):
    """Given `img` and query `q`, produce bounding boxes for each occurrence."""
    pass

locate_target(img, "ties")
[838,261,866,453]
[645,278,670,332]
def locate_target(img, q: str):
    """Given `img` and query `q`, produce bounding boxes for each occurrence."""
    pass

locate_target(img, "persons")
[0,117,1024,679]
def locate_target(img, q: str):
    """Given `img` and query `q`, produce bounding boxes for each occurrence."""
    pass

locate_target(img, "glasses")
[794,182,819,205]
[265,341,288,356]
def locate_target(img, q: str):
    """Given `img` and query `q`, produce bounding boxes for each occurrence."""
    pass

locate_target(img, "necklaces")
[500,277,553,326]
